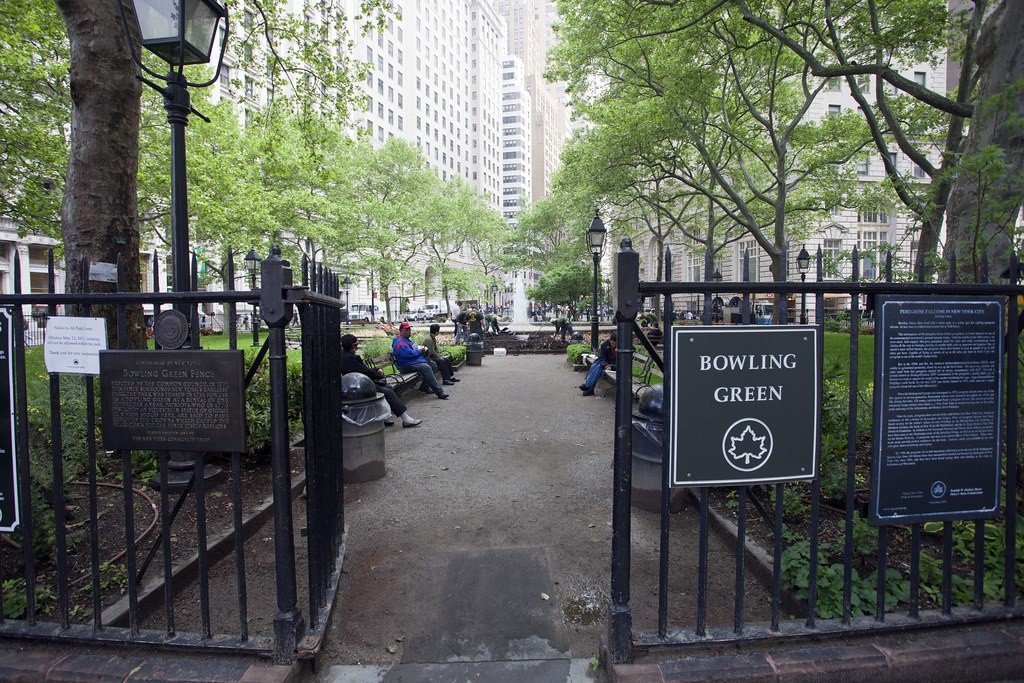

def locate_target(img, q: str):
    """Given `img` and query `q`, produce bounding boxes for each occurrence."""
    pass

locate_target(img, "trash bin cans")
[341,371,388,483]
[570,332,584,344]
[629,384,687,514]
[466,333,482,366]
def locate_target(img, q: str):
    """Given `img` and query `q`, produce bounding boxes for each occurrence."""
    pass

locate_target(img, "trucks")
[424,304,452,320]
[749,299,773,325]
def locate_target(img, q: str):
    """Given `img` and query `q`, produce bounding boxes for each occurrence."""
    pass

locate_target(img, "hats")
[400,322,413,329]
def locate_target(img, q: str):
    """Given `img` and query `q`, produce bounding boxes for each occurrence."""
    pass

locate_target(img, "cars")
[406,309,427,321]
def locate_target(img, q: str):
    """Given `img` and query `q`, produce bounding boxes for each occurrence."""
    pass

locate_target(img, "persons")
[673,309,693,320]
[550,317,573,340]
[292,310,299,326]
[422,324,460,385]
[340,334,422,428]
[391,322,449,400]
[453,311,500,342]
[578,332,618,396]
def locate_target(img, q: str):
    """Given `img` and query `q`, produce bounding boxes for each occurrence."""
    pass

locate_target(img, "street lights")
[711,268,723,324]
[491,279,498,314]
[584,208,607,353]
[242,246,264,348]
[342,277,352,330]
[796,244,811,324]
[132,1,225,496]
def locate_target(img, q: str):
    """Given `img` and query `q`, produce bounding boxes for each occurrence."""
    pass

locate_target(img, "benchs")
[579,347,664,404]
[361,349,453,398]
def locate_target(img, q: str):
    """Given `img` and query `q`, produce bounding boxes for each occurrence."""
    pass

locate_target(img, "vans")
[349,303,388,324]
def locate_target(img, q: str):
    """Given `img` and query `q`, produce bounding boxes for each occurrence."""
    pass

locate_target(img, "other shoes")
[402,419,422,428]
[450,377,460,382]
[419,386,434,394]
[583,389,594,396]
[438,392,449,399]
[579,384,589,391]
[443,380,454,385]
[384,421,394,426]
